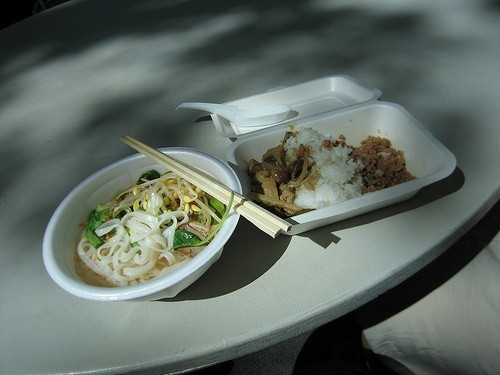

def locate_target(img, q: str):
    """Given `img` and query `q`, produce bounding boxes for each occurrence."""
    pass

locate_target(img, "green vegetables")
[86,189,234,252]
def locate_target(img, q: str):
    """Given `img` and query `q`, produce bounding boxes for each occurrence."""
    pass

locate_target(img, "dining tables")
[0,0,500,375]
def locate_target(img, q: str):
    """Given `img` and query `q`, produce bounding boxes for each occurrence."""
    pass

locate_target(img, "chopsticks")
[119,135,292,239]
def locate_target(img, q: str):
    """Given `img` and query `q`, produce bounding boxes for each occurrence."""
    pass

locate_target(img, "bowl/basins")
[41,147,243,302]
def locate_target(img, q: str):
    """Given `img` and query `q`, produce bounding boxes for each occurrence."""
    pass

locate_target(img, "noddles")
[77,170,213,287]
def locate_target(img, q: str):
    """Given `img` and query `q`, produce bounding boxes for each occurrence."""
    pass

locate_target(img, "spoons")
[177,102,290,125]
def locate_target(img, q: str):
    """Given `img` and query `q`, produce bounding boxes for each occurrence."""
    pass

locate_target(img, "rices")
[284,126,407,212]
